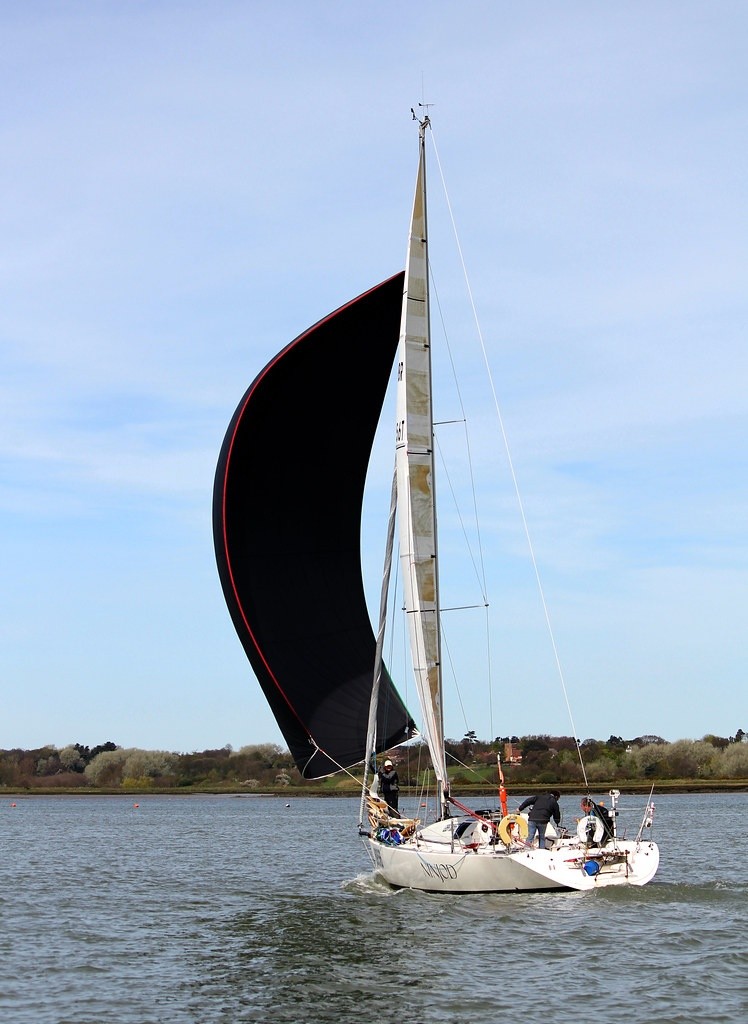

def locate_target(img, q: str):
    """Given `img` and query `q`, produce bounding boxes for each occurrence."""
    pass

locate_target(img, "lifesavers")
[576,814,606,843]
[498,813,530,846]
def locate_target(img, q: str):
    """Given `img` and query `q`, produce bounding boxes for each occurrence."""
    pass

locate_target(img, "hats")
[385,760,393,766]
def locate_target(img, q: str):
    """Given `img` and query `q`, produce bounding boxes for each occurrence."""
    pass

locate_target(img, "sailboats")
[211,103,662,898]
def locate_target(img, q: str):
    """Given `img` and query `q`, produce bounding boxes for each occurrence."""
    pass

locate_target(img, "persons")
[515,790,560,849]
[580,797,614,847]
[369,759,400,818]
